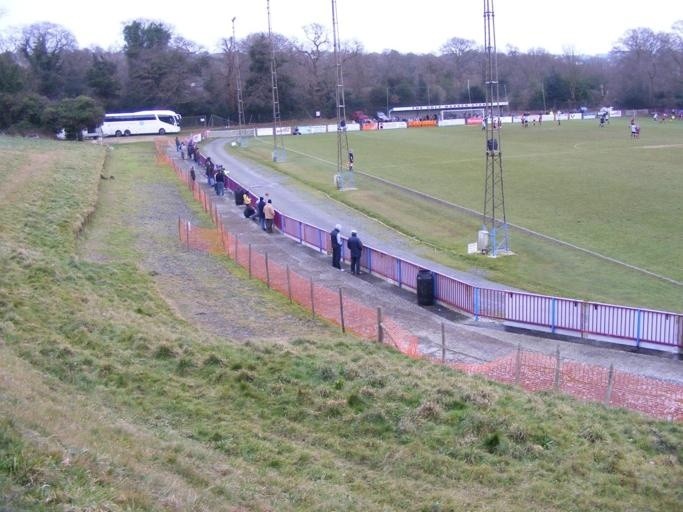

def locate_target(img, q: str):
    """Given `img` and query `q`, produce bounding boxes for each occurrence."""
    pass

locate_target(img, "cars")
[352,111,386,123]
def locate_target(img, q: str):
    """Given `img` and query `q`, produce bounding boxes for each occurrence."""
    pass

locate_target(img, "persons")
[347,149,354,172]
[653,112,682,123]
[347,231,363,276]
[262,199,275,234]
[234,188,259,222]
[203,156,229,197]
[630,123,636,141]
[497,117,502,130]
[174,136,198,163]
[257,196,265,230]
[480,120,485,135]
[635,125,640,142]
[493,120,497,132]
[412,111,495,122]
[329,224,343,270]
[627,117,634,130]
[558,114,560,127]
[190,165,195,181]
[519,113,543,128]
[599,116,605,128]
[604,112,608,122]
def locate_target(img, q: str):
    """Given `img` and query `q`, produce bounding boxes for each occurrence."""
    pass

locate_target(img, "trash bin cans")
[417,269,434,306]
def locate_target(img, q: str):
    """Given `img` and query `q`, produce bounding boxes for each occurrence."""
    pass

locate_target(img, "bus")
[102,110,182,137]
